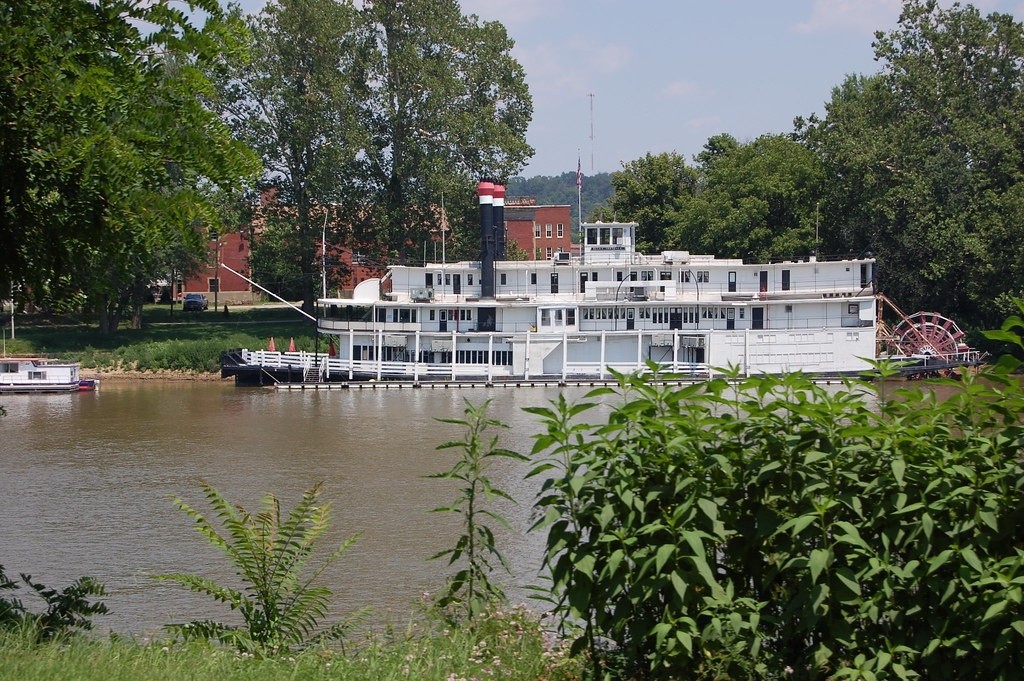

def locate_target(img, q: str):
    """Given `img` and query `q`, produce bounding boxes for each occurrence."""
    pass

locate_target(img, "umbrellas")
[269,337,275,352]
[289,337,296,351]
[328,334,336,358]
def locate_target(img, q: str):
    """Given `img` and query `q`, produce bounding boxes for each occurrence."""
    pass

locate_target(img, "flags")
[576,155,583,204]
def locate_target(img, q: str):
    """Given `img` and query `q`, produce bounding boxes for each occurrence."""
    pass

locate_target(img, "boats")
[0,357,101,394]
[218,174,988,397]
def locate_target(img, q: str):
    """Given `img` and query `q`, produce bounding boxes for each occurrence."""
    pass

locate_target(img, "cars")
[183,293,209,311]
